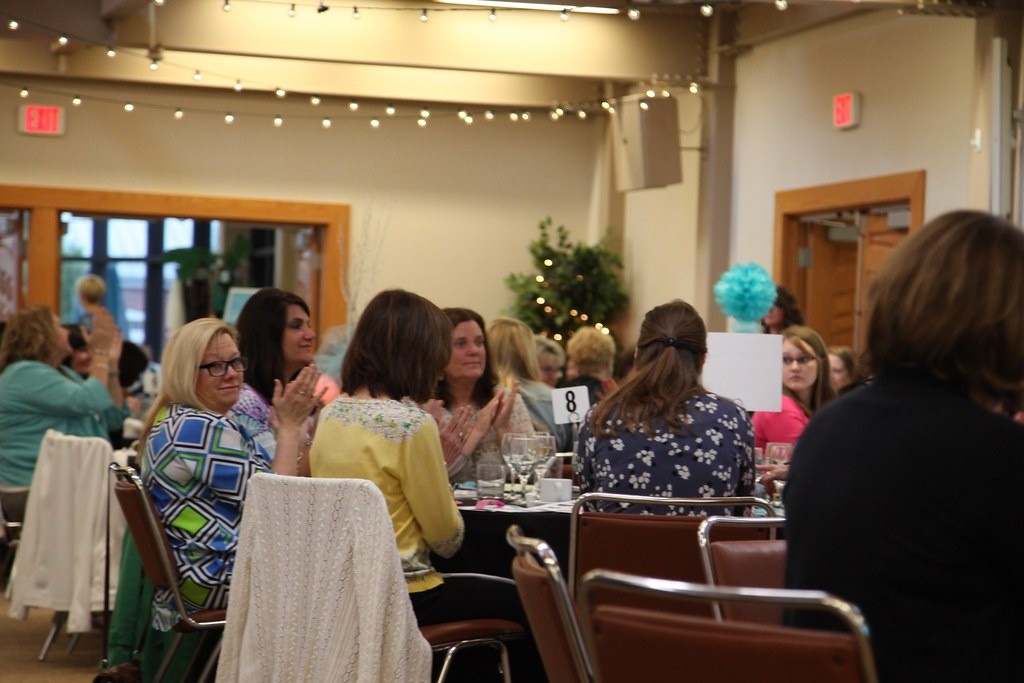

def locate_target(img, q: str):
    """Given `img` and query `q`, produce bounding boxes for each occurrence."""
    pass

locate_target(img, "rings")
[298,389,306,395]
[458,432,465,440]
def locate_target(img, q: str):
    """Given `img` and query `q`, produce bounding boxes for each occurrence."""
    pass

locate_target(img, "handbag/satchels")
[92,662,141,683]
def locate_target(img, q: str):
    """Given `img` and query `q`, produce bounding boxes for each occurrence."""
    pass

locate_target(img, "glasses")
[195,357,248,377]
[783,357,816,364]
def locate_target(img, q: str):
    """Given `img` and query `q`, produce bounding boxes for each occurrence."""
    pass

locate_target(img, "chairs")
[0,427,880,683]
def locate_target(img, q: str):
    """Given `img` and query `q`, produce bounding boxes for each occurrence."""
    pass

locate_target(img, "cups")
[540,478,573,502]
[754,447,762,477]
[475,462,506,500]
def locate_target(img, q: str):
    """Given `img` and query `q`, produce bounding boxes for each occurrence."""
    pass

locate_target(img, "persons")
[762,287,801,333]
[781,210,1024,683]
[0,269,616,683]
[820,345,855,391]
[577,303,754,517]
[750,323,825,461]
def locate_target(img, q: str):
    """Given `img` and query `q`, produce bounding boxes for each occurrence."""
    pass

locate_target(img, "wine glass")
[526,431,556,498]
[511,438,539,505]
[765,443,795,495]
[500,433,528,500]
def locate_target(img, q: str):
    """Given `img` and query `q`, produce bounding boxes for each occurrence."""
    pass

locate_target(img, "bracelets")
[92,349,108,356]
[88,363,109,371]
